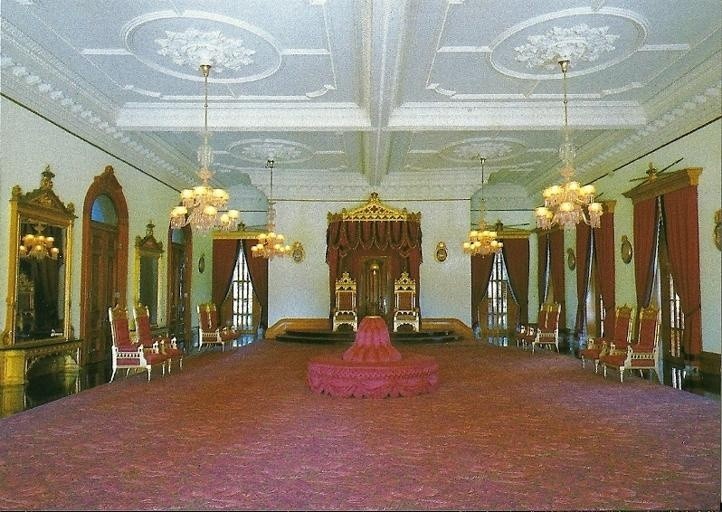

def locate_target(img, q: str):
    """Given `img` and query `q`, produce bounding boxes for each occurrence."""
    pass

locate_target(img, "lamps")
[17,220,62,263]
[530,58,609,234]
[250,158,293,262]
[166,63,243,235]
[462,155,504,259]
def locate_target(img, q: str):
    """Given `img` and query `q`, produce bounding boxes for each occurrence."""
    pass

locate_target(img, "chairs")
[391,271,421,334]
[207,301,241,350]
[196,302,232,353]
[107,303,168,385]
[620,303,662,384]
[581,305,620,375]
[524,301,561,354]
[332,271,358,334]
[600,302,635,381]
[132,302,185,375]
[515,302,553,350]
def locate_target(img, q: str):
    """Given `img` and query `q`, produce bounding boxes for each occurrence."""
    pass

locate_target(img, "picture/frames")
[563,246,577,271]
[619,234,633,265]
[289,240,306,265]
[712,209,722,252]
[433,241,451,264]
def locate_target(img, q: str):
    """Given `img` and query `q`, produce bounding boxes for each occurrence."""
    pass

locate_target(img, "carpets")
[0,335,721,511]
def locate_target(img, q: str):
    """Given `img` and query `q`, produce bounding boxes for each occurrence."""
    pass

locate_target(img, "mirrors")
[132,222,165,327]
[6,162,79,345]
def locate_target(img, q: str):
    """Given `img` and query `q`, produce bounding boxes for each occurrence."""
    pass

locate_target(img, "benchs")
[304,347,440,399]
